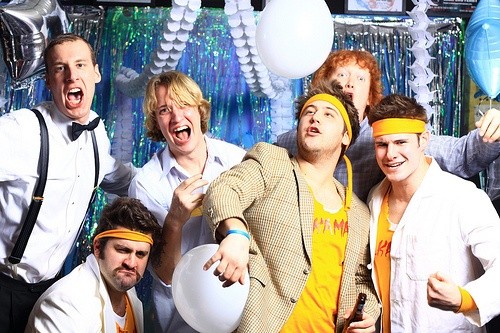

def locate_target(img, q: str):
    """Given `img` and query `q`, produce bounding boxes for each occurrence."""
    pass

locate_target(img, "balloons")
[464,0,500,99]
[254,0,335,80]
[0,1,68,89]
[171,244,251,333]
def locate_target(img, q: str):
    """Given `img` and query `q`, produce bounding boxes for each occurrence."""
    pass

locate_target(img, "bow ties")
[72,117,100,141]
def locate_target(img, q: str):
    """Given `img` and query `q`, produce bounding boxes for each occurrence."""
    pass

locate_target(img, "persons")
[368,0,395,11]
[272,50,500,204]
[25,198,161,333]
[203,79,378,333]
[365,93,500,333]
[0,33,142,333]
[128,71,249,333]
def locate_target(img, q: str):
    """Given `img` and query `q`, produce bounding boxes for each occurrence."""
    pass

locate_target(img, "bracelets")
[225,229,252,240]
